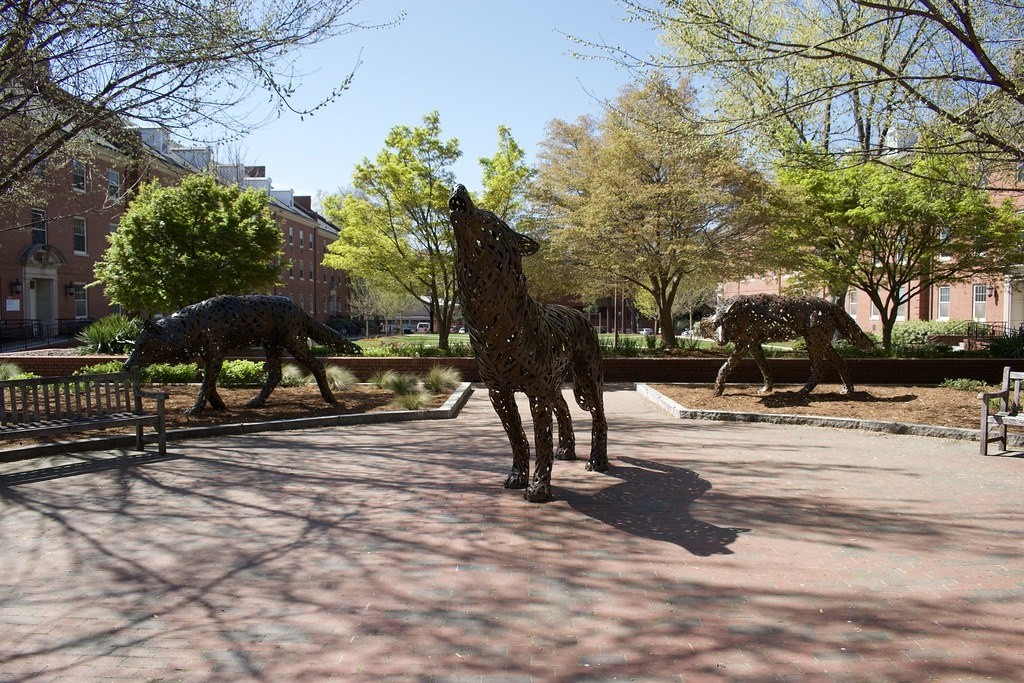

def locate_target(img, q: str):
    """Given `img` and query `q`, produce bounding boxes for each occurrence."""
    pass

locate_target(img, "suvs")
[403,322,430,335]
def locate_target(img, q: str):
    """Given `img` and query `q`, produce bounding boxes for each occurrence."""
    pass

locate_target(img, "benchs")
[977,366,1024,455]
[0,366,169,456]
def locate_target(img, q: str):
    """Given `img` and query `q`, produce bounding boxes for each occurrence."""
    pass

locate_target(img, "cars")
[450,326,466,335]
[387,325,396,333]
[637,328,662,336]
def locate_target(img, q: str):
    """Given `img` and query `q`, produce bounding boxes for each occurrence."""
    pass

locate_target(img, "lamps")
[10,276,21,296]
[986,282,996,297]
[64,281,74,297]
